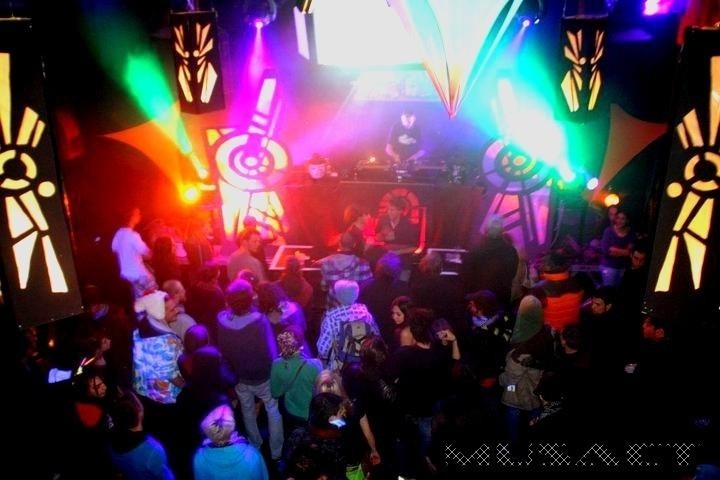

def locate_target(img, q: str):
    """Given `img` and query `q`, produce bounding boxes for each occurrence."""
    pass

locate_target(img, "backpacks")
[338,320,370,364]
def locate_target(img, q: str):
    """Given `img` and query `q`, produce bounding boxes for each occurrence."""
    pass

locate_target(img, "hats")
[135,291,167,320]
[227,280,257,299]
[336,281,359,304]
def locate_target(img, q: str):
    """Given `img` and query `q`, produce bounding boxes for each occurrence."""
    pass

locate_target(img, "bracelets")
[369,448,377,453]
[451,337,457,344]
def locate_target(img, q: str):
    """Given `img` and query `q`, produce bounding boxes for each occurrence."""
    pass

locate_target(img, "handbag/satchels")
[278,395,286,414]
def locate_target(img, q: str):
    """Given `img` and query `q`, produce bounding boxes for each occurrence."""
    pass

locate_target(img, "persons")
[128,291,190,440]
[107,300,144,377]
[254,278,317,367]
[234,213,269,270]
[599,208,634,286]
[280,392,354,478]
[159,275,197,343]
[335,204,377,256]
[104,391,177,480]
[408,251,458,316]
[557,322,590,372]
[111,204,160,303]
[316,233,382,328]
[315,276,380,374]
[622,309,688,424]
[209,230,263,288]
[383,309,464,472]
[76,272,132,349]
[463,289,515,378]
[513,248,584,331]
[390,293,415,351]
[69,326,114,378]
[356,250,417,336]
[457,215,519,310]
[67,371,113,438]
[594,202,621,237]
[617,241,650,300]
[269,323,326,445]
[313,365,383,468]
[177,345,238,474]
[184,263,226,343]
[580,283,628,365]
[372,200,421,250]
[178,322,240,395]
[480,293,560,442]
[215,277,287,470]
[150,235,182,290]
[193,402,269,480]
[277,254,315,312]
[20,325,53,371]
[343,334,400,479]
